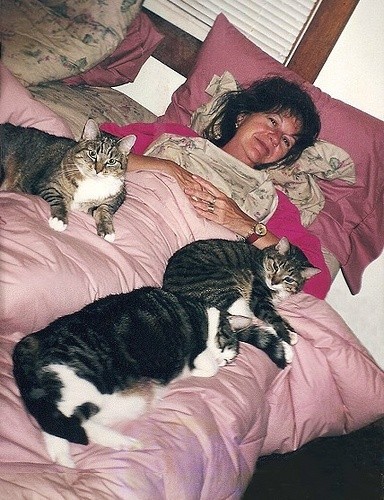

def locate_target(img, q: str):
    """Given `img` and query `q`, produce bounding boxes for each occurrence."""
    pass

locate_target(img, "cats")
[11,286,253,468]
[162,236,321,369]
[0,119,137,242]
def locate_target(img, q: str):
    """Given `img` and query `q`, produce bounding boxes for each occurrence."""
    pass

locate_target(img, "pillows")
[0,0,165,87]
[155,13,384,296]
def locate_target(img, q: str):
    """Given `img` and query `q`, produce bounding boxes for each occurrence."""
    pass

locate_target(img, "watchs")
[245,223,267,245]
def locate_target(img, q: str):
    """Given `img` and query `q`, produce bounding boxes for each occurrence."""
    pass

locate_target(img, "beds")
[1,87,384,500]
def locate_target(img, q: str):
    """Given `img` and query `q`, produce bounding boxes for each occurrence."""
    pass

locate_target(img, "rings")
[211,197,216,205]
[208,206,214,214]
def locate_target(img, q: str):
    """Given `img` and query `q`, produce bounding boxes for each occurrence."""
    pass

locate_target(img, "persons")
[99,74,332,301]
